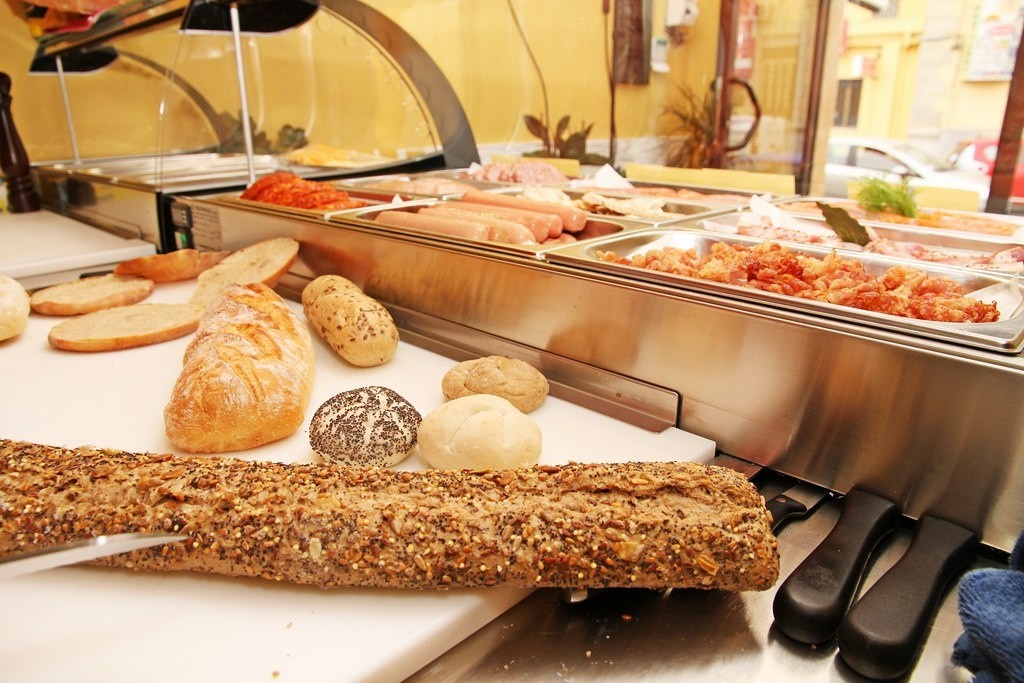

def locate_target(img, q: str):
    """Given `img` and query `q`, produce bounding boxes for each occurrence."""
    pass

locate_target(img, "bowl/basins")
[47,150,1024,354]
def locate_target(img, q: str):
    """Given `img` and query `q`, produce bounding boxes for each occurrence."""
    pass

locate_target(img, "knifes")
[0,533,188,577]
[622,457,979,680]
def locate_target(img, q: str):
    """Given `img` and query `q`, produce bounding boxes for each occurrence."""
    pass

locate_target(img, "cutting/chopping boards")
[0,273,716,682]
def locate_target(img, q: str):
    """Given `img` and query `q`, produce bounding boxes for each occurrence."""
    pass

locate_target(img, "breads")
[0,434,782,591]
[0,236,549,469]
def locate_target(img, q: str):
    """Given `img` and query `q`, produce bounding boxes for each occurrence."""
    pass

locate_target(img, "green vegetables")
[853,173,918,217]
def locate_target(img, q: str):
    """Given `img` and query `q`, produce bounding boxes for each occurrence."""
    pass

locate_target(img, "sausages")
[373,190,590,249]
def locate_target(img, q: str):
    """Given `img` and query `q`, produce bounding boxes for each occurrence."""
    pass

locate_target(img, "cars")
[727,114,1024,217]
